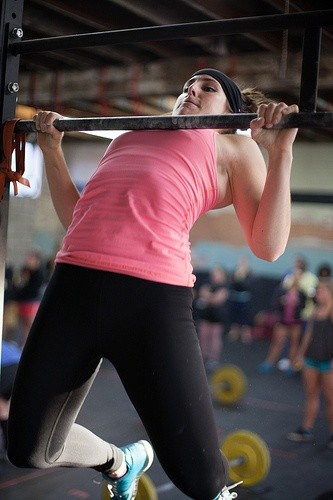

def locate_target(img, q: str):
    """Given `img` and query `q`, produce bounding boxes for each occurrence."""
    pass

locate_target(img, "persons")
[256,259,320,376]
[226,257,256,344]
[2,69,299,500]
[19,252,45,334]
[287,283,333,442]
[200,265,230,371]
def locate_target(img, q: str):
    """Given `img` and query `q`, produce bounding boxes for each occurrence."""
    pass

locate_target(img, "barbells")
[206,362,247,406]
[104,429,272,498]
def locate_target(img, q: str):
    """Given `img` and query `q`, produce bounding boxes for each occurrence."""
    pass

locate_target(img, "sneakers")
[101,439,154,500]
[213,481,243,500]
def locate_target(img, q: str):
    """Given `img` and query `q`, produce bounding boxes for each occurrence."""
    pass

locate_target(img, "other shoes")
[288,426,314,441]
